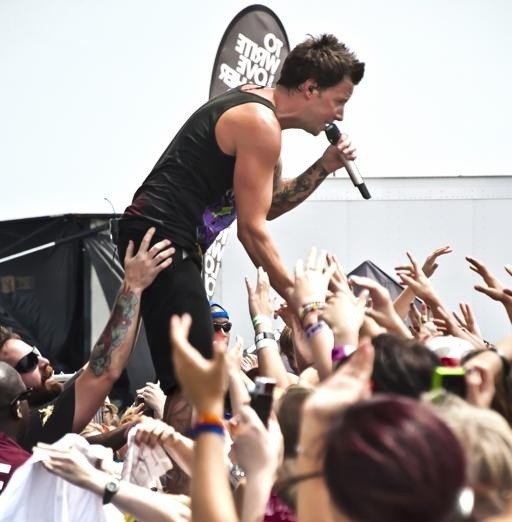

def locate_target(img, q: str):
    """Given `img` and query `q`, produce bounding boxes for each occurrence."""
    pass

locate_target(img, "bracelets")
[193,412,225,430]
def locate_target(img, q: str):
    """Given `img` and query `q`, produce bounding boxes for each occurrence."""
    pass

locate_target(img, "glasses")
[213,323,232,332]
[9,387,33,406]
[102,411,110,416]
[15,345,40,374]
[272,471,325,510]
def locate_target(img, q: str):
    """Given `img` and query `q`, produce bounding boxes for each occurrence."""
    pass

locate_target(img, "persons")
[0,363,32,497]
[0,226,176,453]
[31,246,511,522]
[115,34,358,428]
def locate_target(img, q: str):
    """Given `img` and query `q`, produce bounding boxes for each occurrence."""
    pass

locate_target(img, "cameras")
[433,362,468,399]
[255,378,276,395]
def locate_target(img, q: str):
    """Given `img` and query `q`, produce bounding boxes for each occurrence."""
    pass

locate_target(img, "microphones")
[324,123,372,200]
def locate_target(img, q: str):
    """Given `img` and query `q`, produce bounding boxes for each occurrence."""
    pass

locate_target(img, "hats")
[209,302,228,319]
[423,335,475,377]
[103,395,118,414]
